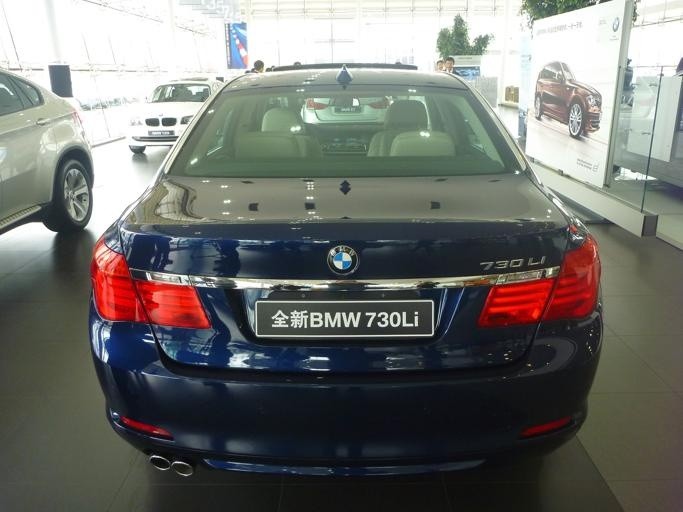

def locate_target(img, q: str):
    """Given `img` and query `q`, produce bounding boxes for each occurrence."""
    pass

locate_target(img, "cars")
[88,64,603,479]
[0,67,94,236]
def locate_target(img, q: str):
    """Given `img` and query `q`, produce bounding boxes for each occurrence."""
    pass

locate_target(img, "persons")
[437,60,445,71]
[442,57,459,75]
[246,60,264,73]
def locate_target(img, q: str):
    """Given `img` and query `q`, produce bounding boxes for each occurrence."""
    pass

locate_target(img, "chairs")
[391,131,455,155]
[236,133,299,159]
[368,99,427,155]
[260,107,322,158]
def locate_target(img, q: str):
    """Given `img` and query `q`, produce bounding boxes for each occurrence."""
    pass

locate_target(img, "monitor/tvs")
[453,65,480,81]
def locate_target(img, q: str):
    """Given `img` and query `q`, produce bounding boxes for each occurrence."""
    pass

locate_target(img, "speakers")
[49,65,72,97]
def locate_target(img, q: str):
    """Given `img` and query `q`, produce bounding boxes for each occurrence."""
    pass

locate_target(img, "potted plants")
[436,14,490,67]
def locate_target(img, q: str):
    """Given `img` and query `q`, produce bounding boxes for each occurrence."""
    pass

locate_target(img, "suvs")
[535,61,602,138]
[127,75,226,154]
